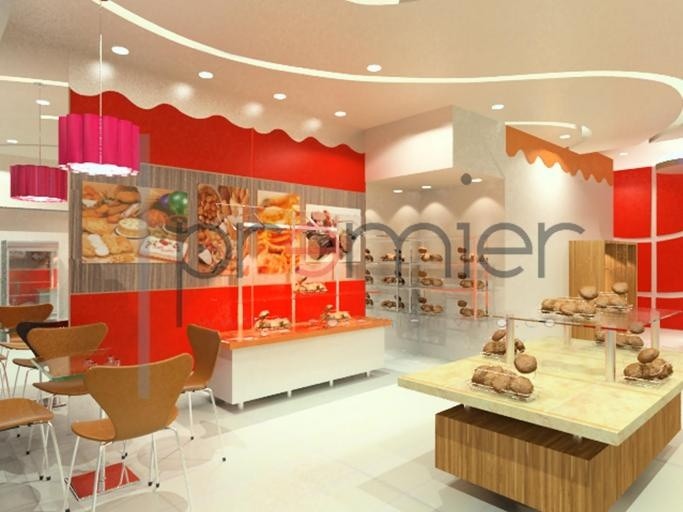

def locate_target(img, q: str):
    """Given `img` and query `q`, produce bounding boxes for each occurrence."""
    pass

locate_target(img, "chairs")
[0,304,154,511]
[64,353,194,511]
[182,324,226,462]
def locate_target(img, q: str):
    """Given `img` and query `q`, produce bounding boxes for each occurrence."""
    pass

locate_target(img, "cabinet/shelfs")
[396,307,683,511]
[365,236,497,361]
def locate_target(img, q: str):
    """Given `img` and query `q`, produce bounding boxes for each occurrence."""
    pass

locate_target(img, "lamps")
[9,84,67,203]
[57,10,142,178]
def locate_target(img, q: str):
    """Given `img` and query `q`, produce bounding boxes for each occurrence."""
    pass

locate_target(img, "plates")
[163,223,188,235]
[114,227,151,239]
[197,183,225,229]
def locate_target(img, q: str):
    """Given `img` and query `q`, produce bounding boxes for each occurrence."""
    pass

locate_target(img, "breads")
[365,247,673,395]
[80,185,149,263]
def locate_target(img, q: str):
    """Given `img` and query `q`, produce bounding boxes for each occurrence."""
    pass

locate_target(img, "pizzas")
[183,230,226,275]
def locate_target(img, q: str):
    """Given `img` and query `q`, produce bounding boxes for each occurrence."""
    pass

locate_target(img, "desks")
[211,317,392,411]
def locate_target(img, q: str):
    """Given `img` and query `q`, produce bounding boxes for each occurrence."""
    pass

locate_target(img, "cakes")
[139,235,189,262]
